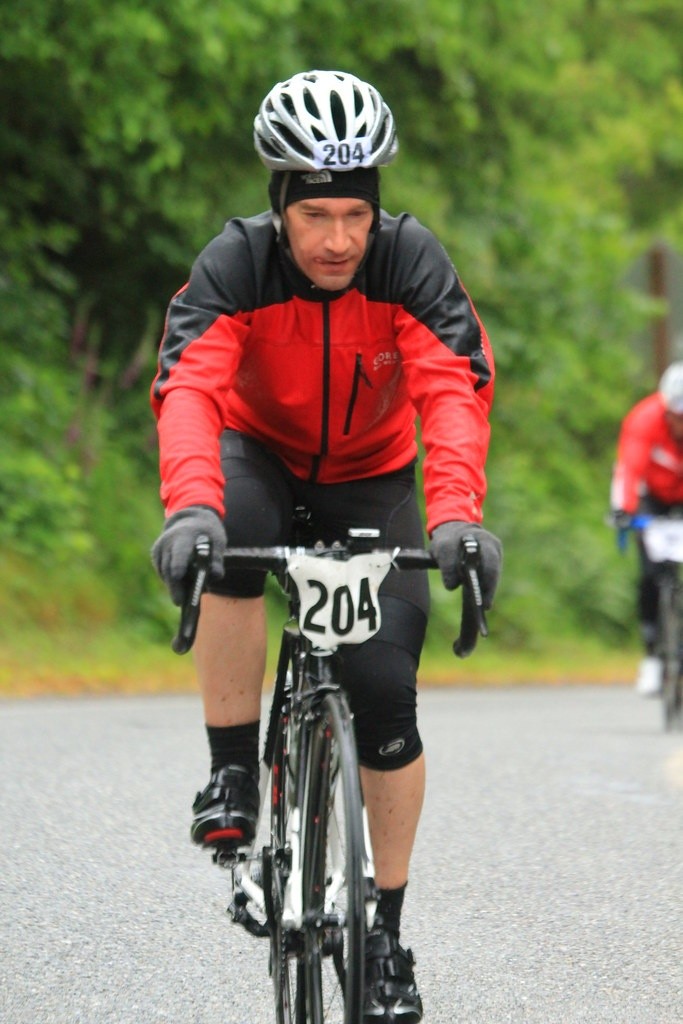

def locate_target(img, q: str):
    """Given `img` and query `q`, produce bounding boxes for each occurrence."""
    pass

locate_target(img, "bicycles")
[611,500,683,727]
[171,493,492,1024]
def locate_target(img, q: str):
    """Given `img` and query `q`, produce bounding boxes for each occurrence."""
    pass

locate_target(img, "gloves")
[149,505,227,607]
[429,521,502,612]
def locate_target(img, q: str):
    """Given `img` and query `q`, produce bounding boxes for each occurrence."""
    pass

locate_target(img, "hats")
[268,167,381,211]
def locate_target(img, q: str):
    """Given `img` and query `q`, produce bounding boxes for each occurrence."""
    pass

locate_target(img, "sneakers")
[190,760,258,849]
[358,926,423,1024]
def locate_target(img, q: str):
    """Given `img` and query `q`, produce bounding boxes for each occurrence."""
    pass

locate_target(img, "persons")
[609,360,683,694]
[148,69,499,1023]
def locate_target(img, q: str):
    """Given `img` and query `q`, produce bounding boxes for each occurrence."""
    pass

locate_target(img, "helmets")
[659,360,683,413]
[253,71,399,172]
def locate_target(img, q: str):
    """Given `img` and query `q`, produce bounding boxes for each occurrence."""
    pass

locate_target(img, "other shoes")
[638,655,664,696]
